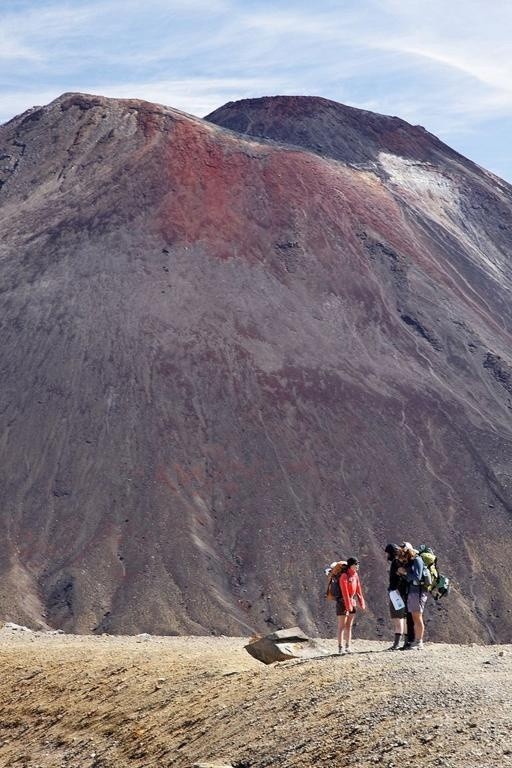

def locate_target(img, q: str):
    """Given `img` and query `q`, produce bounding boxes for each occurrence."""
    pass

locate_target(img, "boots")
[388,634,402,651]
[399,634,410,649]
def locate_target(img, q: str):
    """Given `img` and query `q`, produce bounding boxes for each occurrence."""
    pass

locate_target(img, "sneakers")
[338,645,343,655]
[346,646,351,654]
[409,643,423,649]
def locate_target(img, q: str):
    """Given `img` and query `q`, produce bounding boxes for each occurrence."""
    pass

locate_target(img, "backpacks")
[419,545,449,600]
[326,561,347,600]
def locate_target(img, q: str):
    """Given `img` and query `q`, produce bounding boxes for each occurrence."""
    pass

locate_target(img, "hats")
[385,543,396,552]
[400,542,412,556]
[348,557,360,565]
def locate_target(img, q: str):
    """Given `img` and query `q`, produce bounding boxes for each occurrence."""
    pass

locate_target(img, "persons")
[384,541,428,651]
[335,557,365,655]
